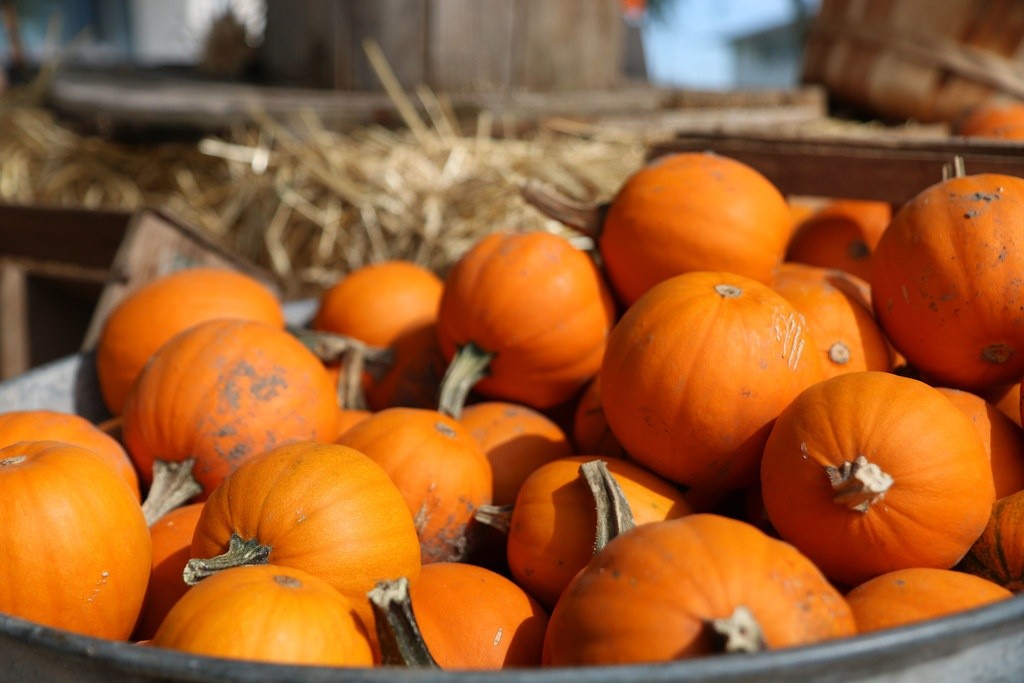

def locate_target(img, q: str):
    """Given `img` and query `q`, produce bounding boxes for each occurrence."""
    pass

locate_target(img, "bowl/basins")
[0,236,1024,683]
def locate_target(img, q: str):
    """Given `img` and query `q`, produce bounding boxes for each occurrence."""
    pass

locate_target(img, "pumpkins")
[0,99,1024,675]
